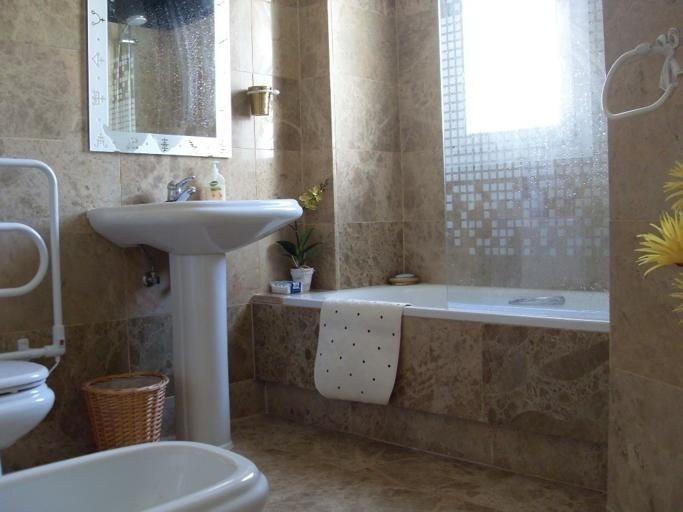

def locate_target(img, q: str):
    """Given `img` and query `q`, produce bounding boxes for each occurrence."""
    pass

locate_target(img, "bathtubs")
[254,277,611,493]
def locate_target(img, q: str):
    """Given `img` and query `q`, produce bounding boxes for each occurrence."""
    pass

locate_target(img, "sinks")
[86,198,305,255]
[1,441,268,512]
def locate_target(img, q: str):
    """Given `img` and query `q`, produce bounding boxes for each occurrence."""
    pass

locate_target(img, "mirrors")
[86,2,232,160]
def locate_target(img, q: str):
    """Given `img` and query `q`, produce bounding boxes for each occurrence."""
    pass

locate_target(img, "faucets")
[165,174,196,202]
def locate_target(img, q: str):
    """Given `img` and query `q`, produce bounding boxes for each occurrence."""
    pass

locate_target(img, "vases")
[291,265,314,293]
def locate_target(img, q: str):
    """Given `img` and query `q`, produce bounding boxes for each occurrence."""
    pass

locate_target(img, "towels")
[314,296,410,406]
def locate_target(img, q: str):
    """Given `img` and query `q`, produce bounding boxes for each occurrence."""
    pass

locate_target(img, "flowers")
[635,161,683,347]
[275,177,329,268]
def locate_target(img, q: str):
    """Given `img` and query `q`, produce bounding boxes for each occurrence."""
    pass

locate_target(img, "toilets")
[1,357,56,446]
